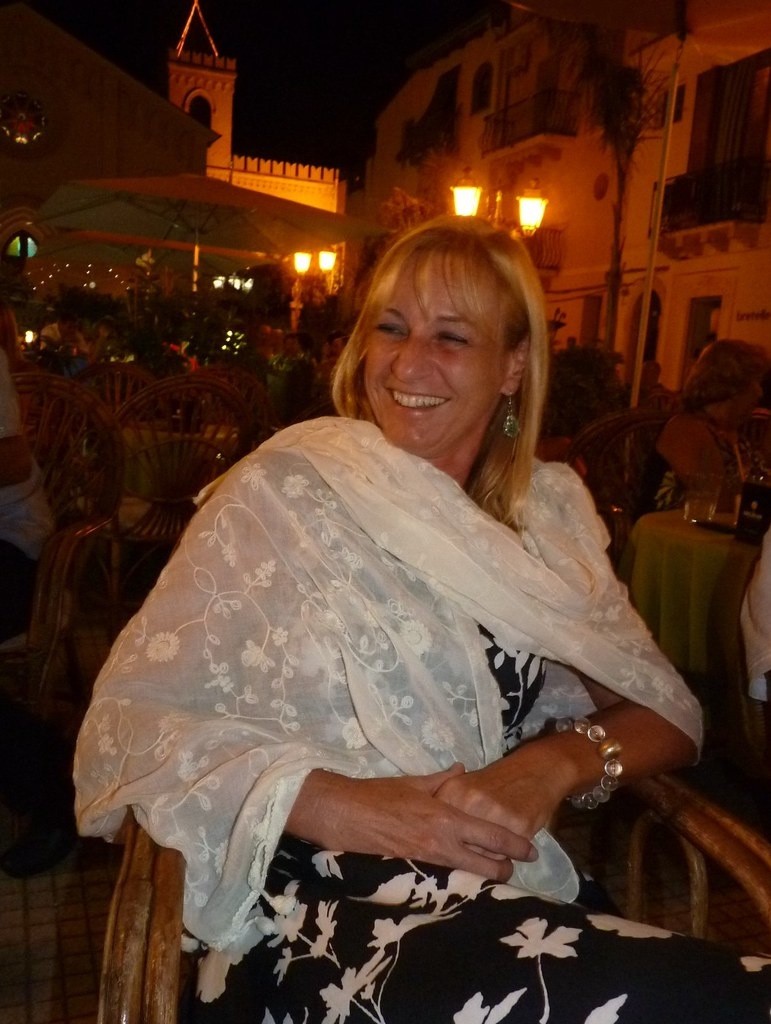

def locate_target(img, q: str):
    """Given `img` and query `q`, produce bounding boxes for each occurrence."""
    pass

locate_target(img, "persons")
[34,292,357,430]
[630,337,771,530]
[74,214,771,1024]
[0,348,52,643]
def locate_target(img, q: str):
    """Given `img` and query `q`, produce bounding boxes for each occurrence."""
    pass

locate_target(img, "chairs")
[560,409,674,577]
[71,339,350,622]
[0,371,123,723]
[99,772,770,1024]
[736,406,771,469]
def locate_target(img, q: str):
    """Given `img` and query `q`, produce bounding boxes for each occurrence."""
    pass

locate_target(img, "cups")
[684,471,721,521]
[744,475,771,485]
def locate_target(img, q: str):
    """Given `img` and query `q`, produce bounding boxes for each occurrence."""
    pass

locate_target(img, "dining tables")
[113,418,236,498]
[622,510,737,696]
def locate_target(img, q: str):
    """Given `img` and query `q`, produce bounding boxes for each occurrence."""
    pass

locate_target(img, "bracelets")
[554,716,623,811]
